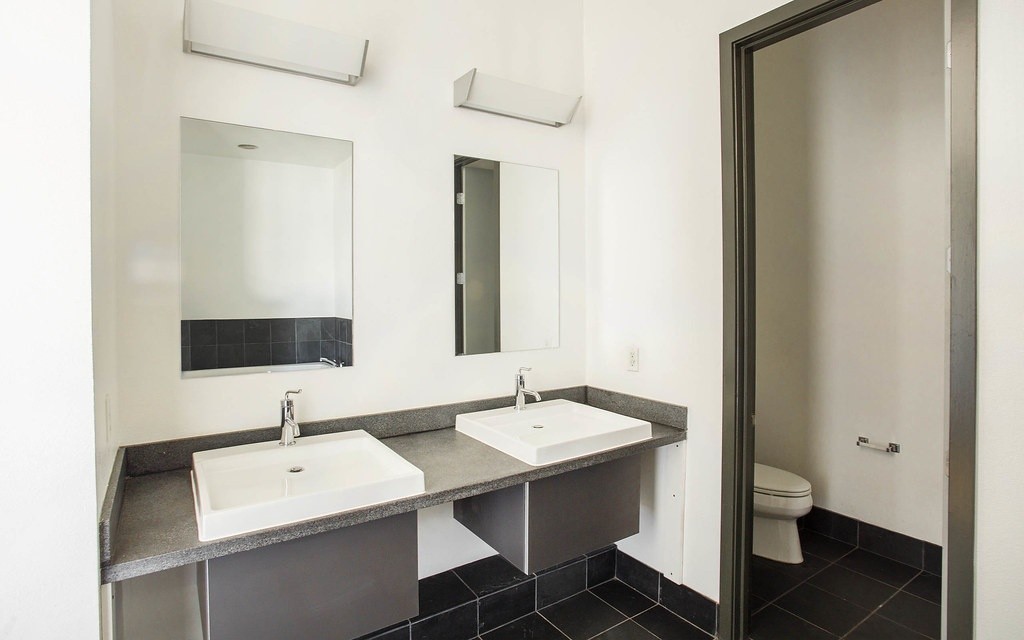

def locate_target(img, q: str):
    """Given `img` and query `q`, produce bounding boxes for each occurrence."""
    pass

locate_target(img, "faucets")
[320,357,343,367]
[515,367,541,409]
[278,389,302,447]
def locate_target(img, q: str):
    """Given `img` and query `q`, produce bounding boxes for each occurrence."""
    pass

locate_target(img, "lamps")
[183,0,369,86]
[454,67,583,127]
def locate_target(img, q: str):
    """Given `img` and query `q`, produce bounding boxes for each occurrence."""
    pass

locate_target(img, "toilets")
[751,463,813,565]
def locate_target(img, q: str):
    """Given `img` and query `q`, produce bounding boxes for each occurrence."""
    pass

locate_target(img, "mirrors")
[454,155,559,356]
[179,117,352,377]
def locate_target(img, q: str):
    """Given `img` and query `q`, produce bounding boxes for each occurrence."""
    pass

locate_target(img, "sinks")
[189,427,426,542]
[456,398,653,466]
